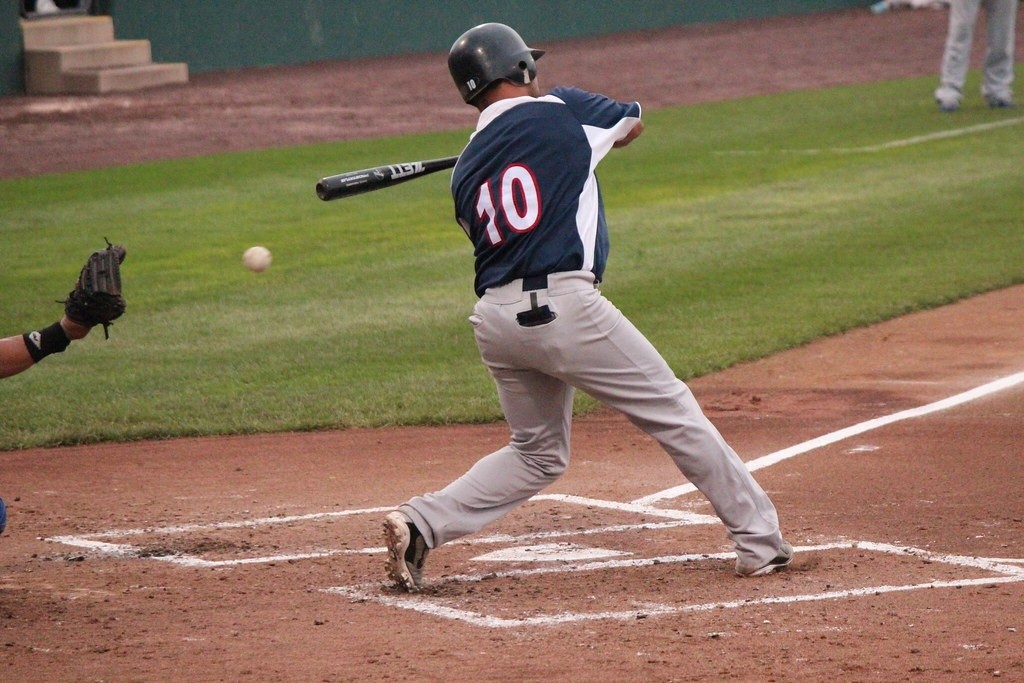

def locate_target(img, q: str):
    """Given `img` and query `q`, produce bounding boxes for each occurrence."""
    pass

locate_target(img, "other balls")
[239,242,274,275]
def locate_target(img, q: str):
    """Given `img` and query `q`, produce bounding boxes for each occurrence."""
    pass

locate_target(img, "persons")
[0,241,127,535]
[381,23,790,593]
[936,0,1017,112]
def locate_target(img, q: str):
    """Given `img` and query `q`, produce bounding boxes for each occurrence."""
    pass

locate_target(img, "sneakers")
[731,539,795,578]
[380,511,429,596]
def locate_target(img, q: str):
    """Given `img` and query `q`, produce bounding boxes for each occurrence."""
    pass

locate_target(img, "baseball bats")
[313,153,458,203]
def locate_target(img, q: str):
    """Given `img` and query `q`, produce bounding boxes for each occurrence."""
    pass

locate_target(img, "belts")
[522,274,547,294]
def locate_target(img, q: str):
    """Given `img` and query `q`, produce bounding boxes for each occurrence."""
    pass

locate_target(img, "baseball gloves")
[53,234,129,344]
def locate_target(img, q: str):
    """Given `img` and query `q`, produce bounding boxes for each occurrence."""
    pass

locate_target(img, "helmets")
[447,20,547,104]
